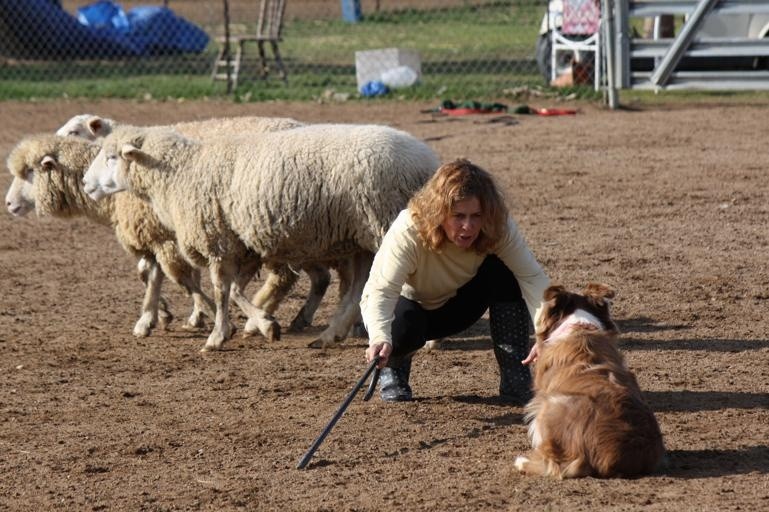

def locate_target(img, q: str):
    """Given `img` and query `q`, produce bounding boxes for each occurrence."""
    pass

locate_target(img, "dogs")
[512,280,670,482]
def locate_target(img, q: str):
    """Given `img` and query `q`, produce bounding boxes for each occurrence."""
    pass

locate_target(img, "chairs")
[546,1,602,94]
[205,1,288,94]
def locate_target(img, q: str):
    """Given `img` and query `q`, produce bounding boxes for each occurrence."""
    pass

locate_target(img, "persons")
[358,158,557,405]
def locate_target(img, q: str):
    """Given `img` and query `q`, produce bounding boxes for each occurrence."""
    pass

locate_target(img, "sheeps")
[81,123,443,355]
[4,132,369,339]
[55,113,312,309]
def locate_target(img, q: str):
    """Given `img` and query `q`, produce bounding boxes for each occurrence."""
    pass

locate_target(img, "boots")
[488,299,533,405]
[379,335,426,401]
